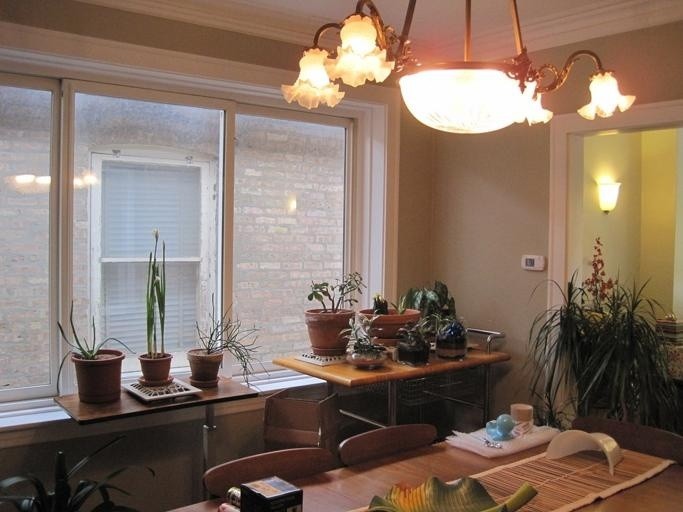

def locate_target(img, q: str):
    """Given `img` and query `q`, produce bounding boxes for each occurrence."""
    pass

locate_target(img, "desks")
[273,327,511,428]
[167,417,683,512]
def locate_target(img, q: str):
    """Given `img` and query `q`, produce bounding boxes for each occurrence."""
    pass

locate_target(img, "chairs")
[204,447,334,497]
[338,424,437,467]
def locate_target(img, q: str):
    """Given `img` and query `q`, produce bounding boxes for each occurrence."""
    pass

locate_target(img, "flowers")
[581,236,620,310]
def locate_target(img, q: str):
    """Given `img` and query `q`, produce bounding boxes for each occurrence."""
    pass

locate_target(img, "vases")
[582,309,611,329]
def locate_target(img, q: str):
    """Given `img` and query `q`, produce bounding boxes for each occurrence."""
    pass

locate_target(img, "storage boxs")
[263,388,339,453]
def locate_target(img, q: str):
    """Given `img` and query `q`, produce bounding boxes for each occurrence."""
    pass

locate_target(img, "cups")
[511,403,533,435]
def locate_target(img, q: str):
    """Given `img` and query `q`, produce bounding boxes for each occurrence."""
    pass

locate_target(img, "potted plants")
[520,265,680,430]
[337,309,388,369]
[432,313,467,359]
[357,293,421,339]
[139,229,173,381]
[187,293,271,392]
[304,272,367,356]
[56,300,134,405]
[396,316,431,364]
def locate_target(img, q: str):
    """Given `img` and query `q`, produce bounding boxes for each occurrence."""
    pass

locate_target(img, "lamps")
[281,0,636,133]
[597,183,621,214]
